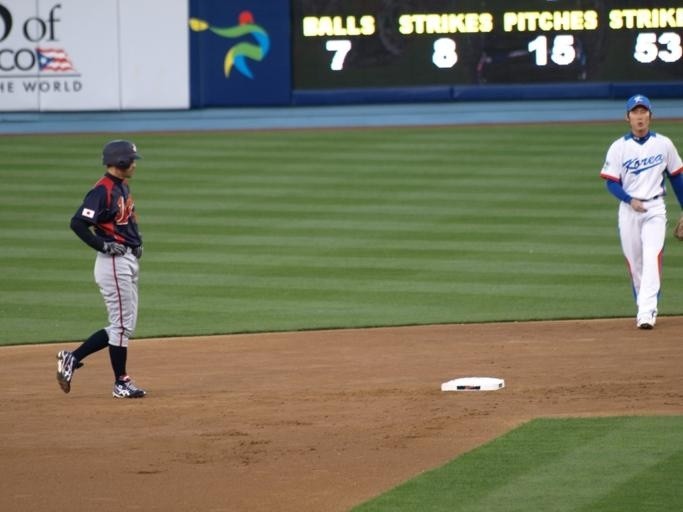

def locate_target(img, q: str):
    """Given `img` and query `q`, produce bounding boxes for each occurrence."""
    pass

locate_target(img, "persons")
[57,139,146,398]
[600,94,683,329]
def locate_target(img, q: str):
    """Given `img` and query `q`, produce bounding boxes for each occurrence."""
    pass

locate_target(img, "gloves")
[107,241,127,255]
[133,245,142,259]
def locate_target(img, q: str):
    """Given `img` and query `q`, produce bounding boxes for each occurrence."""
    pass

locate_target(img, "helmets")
[102,140,142,166]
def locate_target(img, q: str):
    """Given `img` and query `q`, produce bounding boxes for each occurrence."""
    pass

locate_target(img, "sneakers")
[56,349,84,394]
[637,307,657,329]
[112,377,147,399]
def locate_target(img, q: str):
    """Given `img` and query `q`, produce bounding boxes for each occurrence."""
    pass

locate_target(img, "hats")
[626,94,651,112]
